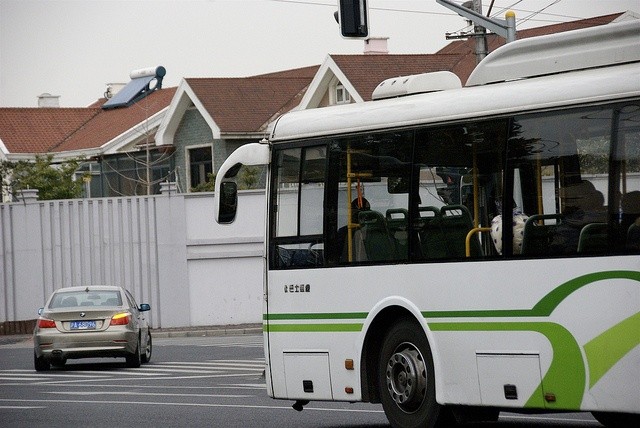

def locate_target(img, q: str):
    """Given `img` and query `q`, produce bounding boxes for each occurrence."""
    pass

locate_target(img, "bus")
[214,20,640,428]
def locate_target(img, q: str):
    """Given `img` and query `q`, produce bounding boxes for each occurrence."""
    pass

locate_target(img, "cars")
[33,286,152,371]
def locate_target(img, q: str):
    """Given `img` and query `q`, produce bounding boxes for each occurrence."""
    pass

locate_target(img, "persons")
[332,197,371,262]
[547,180,606,253]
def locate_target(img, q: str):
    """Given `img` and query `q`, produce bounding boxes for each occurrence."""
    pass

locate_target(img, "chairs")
[439,205,484,260]
[411,206,441,259]
[577,222,609,254]
[521,214,567,256]
[386,208,414,260]
[354,211,392,261]
